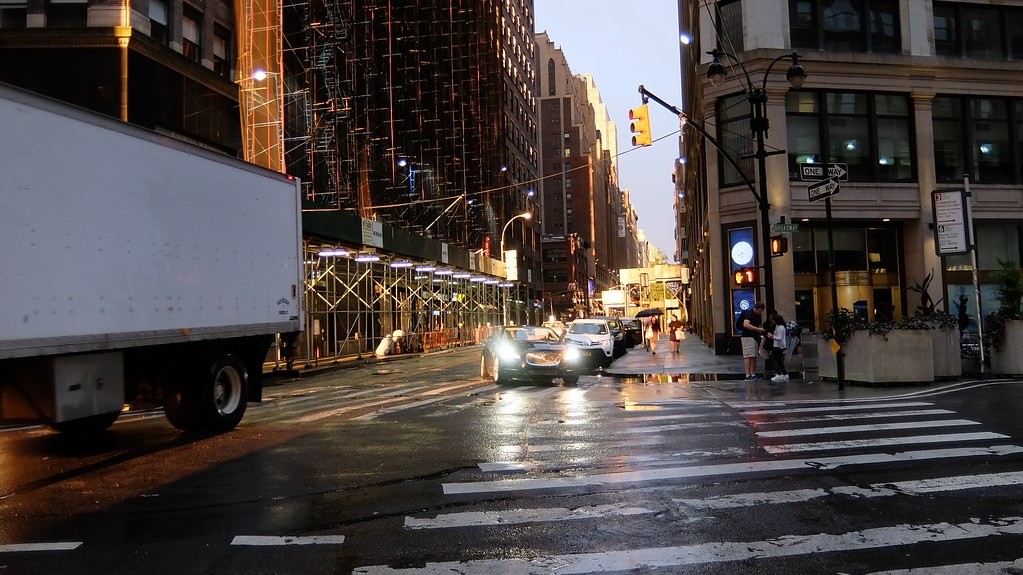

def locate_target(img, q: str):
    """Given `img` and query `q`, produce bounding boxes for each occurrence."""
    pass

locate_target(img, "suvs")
[563,318,619,366]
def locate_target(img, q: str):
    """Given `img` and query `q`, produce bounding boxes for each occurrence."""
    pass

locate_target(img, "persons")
[668,314,683,353]
[279,332,298,369]
[646,316,661,355]
[741,302,789,382]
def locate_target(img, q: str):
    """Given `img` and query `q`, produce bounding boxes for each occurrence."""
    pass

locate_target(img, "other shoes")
[771,374,789,382]
[745,375,763,381]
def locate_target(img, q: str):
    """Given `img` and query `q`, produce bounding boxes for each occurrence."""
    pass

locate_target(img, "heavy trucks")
[0,90,305,434]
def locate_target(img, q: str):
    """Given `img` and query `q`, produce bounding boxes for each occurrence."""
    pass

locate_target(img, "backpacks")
[736,309,751,331]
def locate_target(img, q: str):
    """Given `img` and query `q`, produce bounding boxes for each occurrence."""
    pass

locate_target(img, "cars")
[594,317,628,357]
[481,323,580,385]
[541,322,565,337]
[621,318,642,344]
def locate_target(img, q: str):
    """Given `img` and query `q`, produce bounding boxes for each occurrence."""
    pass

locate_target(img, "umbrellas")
[635,308,664,318]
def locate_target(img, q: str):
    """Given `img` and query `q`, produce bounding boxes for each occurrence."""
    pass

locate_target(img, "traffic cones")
[394,339,402,354]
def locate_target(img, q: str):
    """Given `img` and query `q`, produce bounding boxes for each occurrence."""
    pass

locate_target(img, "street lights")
[609,285,625,291]
[628,287,637,318]
[706,48,806,379]
[500,212,532,326]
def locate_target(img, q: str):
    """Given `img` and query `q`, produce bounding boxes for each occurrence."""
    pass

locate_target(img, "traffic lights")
[771,236,789,255]
[735,268,758,287]
[629,106,653,147]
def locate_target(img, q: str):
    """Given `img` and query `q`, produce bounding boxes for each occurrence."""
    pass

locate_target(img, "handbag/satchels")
[645,326,653,339]
[675,330,685,340]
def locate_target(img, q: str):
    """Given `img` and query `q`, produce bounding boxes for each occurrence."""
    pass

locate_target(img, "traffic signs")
[808,175,839,203]
[799,161,849,183]
